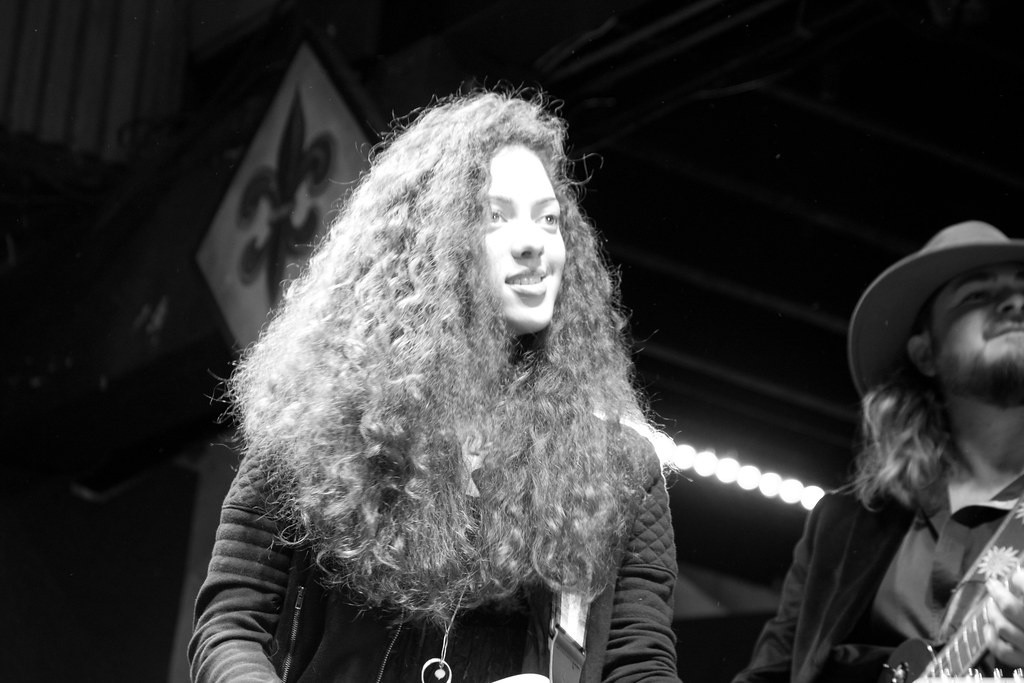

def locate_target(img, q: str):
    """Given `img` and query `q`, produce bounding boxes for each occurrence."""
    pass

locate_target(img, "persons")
[736,224,1024,682]
[186,92,680,683]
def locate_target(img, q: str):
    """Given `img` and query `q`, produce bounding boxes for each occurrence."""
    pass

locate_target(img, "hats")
[847,222,1024,397]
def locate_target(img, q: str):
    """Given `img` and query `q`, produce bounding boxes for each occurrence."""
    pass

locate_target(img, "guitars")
[880,555,1024,683]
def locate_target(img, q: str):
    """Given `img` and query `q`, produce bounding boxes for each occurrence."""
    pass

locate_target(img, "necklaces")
[422,579,467,683]
